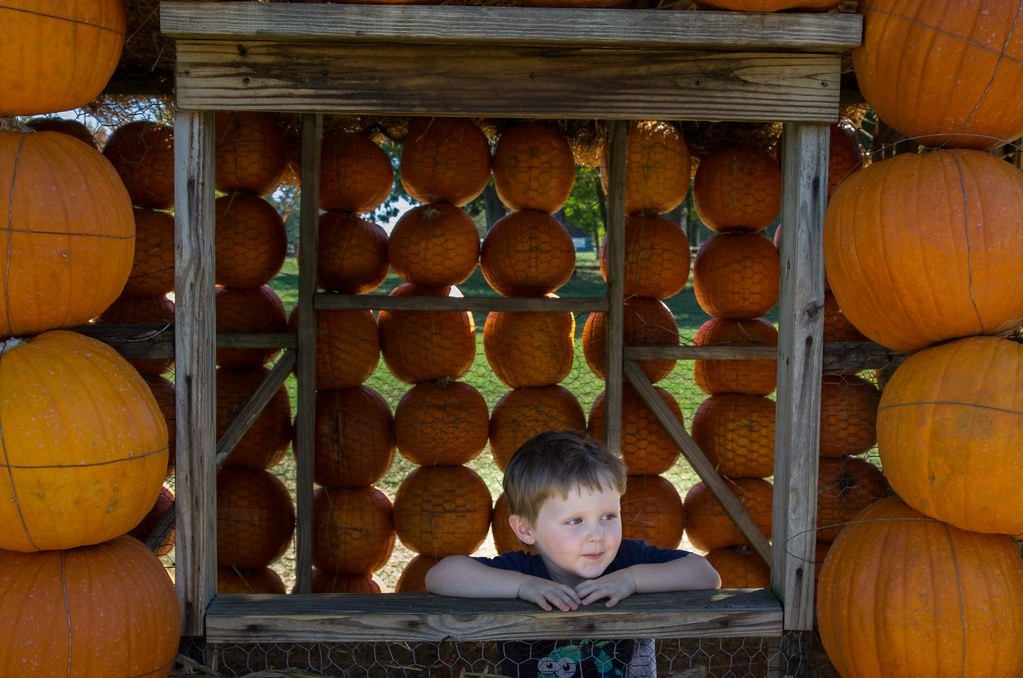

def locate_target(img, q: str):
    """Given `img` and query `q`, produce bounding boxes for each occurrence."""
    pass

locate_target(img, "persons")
[426,429,725,677]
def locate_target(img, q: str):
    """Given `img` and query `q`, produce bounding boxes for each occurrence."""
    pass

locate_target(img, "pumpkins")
[0,0,1021,678]
